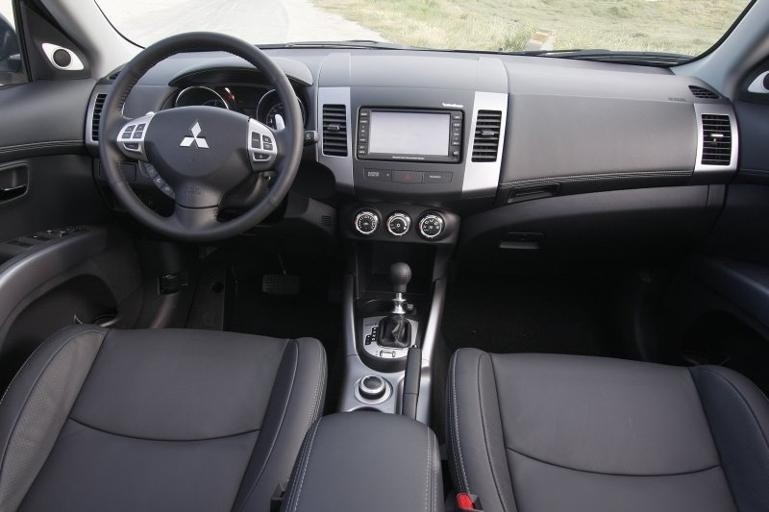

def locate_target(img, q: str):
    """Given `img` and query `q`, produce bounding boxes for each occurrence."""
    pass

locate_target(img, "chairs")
[447,347,768,512]
[0,321,328,512]
